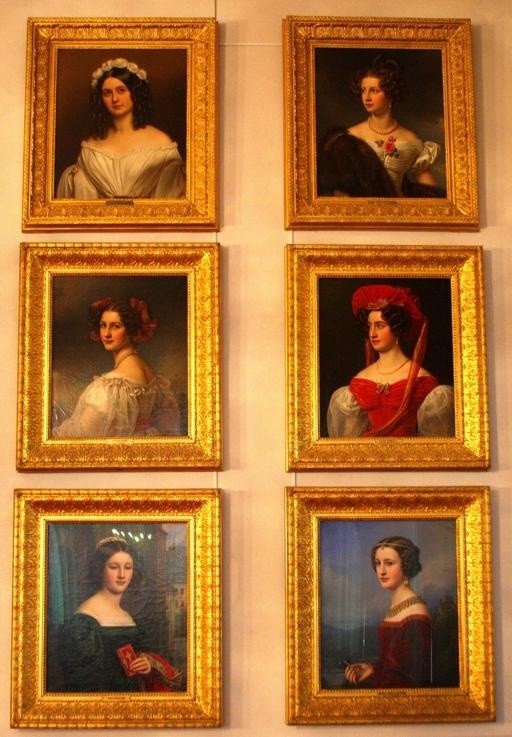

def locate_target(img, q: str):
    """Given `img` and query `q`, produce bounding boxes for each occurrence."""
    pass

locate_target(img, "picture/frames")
[282,483,499,728]
[13,240,225,475]
[6,484,228,733]
[282,241,494,475]
[280,11,483,234]
[18,13,223,236]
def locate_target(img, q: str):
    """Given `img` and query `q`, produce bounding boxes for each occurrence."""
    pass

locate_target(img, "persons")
[52,295,182,437]
[56,55,188,199]
[326,281,456,436]
[58,536,183,691]
[315,54,446,196]
[342,536,439,688]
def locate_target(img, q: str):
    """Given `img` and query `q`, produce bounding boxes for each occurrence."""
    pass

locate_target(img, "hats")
[347,282,429,342]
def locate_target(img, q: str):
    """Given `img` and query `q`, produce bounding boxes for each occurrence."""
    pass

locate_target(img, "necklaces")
[366,115,398,134]
[383,595,426,617]
[113,351,138,370]
[375,358,410,373]
